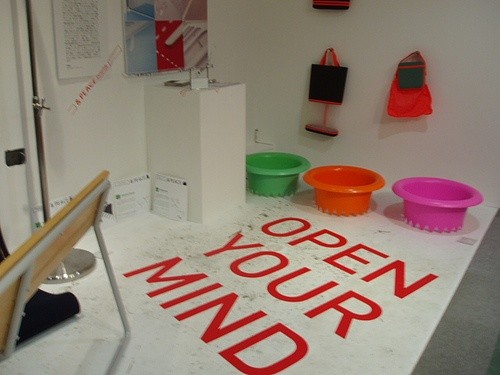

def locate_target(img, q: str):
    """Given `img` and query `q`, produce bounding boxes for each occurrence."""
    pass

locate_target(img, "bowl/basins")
[391,176,484,232]
[246,151,311,197]
[302,164,387,218]
